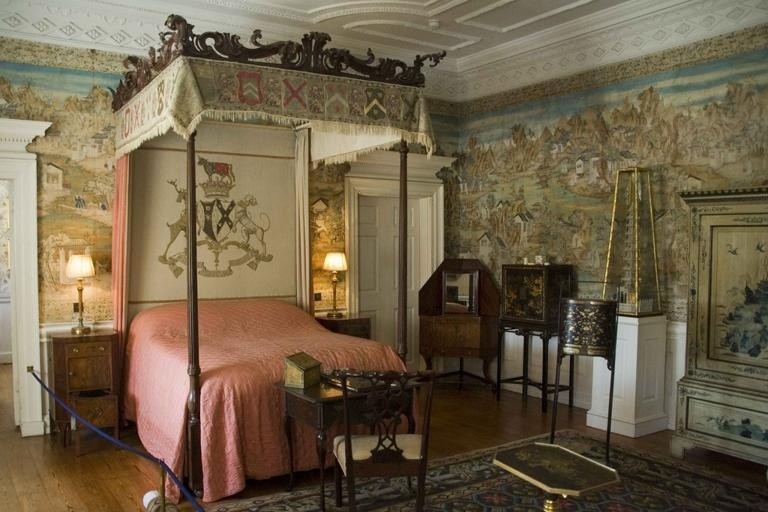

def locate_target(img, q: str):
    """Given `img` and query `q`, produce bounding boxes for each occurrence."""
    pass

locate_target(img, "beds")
[109,13,447,502]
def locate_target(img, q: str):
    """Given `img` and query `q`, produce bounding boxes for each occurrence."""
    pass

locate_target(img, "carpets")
[185,425,768,512]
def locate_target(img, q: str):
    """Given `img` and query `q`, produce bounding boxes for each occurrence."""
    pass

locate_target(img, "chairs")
[331,367,436,512]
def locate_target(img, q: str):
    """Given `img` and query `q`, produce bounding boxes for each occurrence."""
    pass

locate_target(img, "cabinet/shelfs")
[496,264,577,413]
[418,258,502,386]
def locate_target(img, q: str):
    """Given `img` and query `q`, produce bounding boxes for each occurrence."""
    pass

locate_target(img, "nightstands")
[316,316,371,339]
[46,328,121,459]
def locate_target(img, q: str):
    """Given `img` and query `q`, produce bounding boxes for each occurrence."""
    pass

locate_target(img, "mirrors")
[442,269,479,317]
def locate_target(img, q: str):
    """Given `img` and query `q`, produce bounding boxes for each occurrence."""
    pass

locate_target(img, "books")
[321,371,385,392]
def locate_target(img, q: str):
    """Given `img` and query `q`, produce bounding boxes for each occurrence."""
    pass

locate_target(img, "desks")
[274,368,434,512]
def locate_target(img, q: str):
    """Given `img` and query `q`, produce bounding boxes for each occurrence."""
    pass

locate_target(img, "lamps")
[65,254,95,334]
[323,251,348,318]
